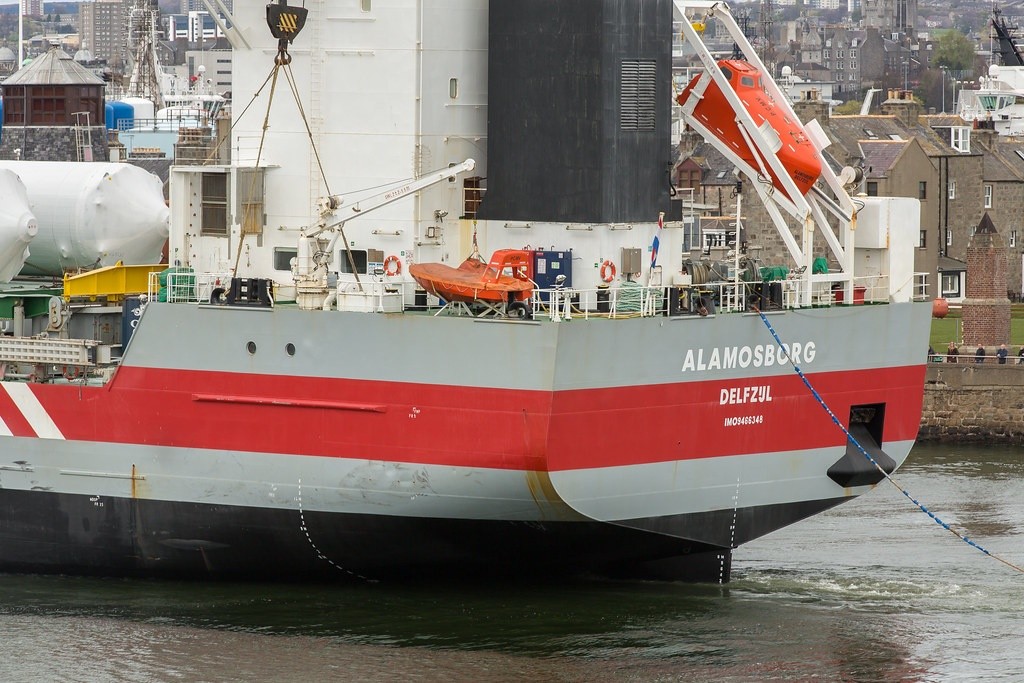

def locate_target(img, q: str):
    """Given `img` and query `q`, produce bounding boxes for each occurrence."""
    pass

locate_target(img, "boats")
[0,0,950,582]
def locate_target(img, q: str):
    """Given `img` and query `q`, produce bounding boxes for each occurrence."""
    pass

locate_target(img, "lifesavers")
[62,364,80,380]
[384,256,402,275]
[600,261,616,281]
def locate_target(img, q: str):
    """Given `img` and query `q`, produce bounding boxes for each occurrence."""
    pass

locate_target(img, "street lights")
[940,65,949,112]
